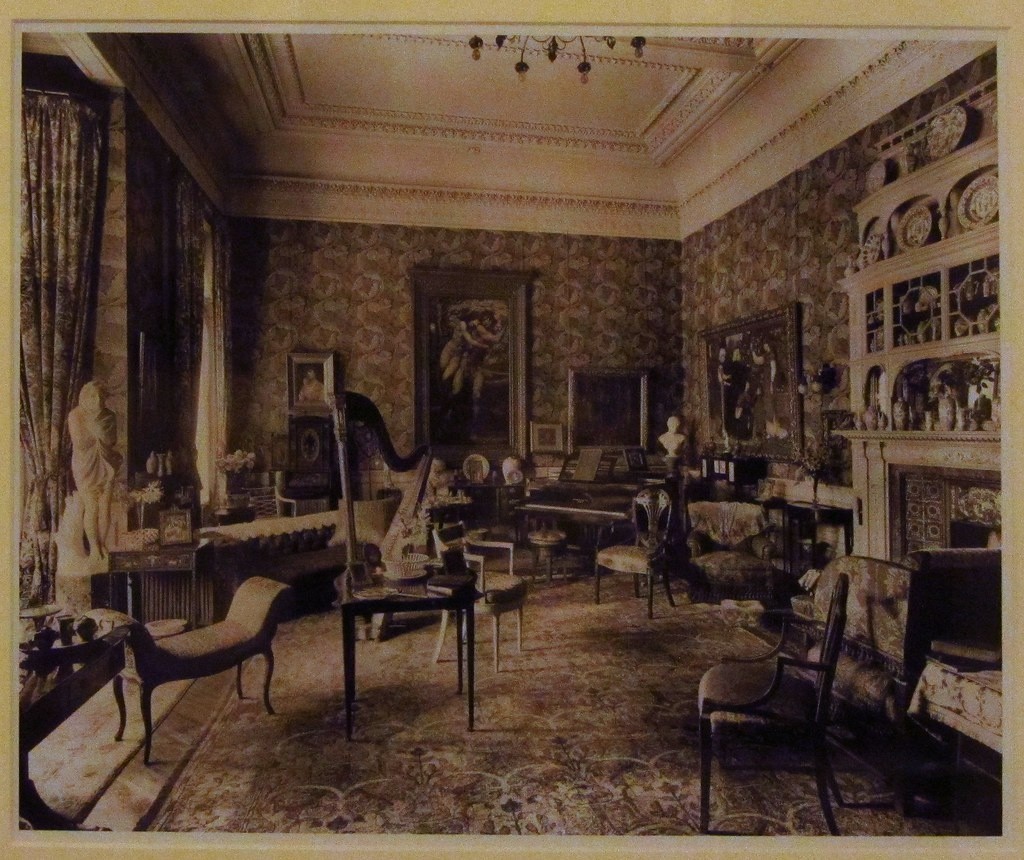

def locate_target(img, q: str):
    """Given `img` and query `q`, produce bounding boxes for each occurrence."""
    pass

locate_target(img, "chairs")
[430,520,526,674]
[593,486,674,619]
[693,571,849,837]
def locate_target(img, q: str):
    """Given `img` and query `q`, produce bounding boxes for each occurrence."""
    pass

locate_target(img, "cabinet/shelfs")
[840,73,1002,435]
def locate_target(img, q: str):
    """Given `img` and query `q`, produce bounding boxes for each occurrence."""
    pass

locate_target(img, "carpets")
[127,542,1003,836]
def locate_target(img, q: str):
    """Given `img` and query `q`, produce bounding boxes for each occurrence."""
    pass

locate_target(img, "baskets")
[381,553,430,579]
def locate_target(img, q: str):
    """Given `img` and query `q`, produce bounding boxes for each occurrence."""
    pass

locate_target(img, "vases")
[853,390,971,432]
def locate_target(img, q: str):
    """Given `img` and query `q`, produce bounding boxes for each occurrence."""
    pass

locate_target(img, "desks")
[106,535,213,634]
[334,564,482,739]
[905,659,1002,835]
[512,498,637,569]
[17,631,125,834]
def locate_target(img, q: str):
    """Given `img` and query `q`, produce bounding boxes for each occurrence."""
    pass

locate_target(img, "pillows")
[326,496,398,548]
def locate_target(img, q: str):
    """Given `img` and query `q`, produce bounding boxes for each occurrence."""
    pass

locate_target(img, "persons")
[659,416,685,454]
[68,382,117,563]
[717,340,777,448]
[299,366,326,404]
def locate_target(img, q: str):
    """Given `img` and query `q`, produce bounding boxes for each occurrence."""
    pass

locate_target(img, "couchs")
[684,500,777,609]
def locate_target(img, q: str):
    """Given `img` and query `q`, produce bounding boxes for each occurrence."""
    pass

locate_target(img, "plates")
[896,206,933,251]
[925,104,968,156]
[865,159,889,193]
[462,454,490,480]
[862,233,883,264]
[957,175,1000,229]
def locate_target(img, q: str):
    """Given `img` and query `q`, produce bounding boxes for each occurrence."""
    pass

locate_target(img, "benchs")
[85,574,294,767]
[207,503,390,618]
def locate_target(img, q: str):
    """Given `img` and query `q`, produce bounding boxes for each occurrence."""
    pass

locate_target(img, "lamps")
[797,359,836,394]
[466,35,649,87]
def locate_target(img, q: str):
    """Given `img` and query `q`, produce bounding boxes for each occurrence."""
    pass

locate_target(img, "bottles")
[854,393,965,430]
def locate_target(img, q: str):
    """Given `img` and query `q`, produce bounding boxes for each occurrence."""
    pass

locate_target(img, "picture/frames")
[411,266,528,464]
[158,508,194,547]
[285,349,336,412]
[622,447,650,473]
[529,420,564,455]
[566,365,649,454]
[696,299,806,467]
